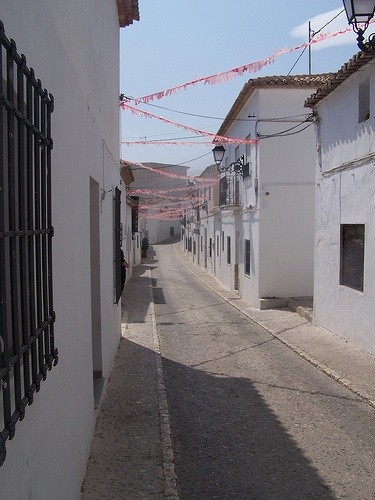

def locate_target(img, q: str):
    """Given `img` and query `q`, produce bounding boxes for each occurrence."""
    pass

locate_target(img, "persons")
[120,240,129,307]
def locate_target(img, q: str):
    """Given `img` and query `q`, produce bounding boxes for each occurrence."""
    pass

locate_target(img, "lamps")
[342,0,374,55]
[212,145,249,177]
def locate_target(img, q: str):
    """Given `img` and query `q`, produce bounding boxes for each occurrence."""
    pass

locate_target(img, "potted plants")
[141,238,149,259]
